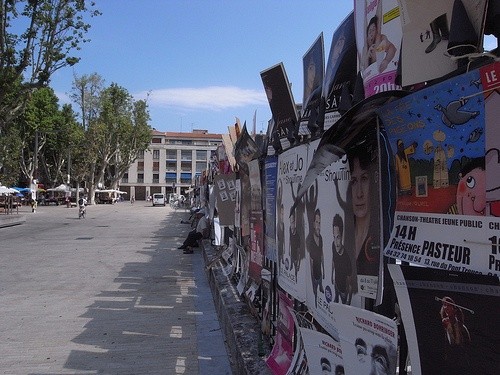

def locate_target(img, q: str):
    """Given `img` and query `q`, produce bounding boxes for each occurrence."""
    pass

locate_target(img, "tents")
[0,182,20,214]
[11,183,127,205]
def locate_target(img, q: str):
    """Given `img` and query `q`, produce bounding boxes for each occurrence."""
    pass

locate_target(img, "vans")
[153,193,165,206]
[71,197,76,207]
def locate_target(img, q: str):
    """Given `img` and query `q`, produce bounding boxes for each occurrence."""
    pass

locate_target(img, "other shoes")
[178,245,187,249]
[183,250,193,254]
[191,244,199,248]
[183,248,189,251]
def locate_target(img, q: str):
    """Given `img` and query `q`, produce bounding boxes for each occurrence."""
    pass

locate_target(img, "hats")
[196,210,204,214]
[194,209,199,213]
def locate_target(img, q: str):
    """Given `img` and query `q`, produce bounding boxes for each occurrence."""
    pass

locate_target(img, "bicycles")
[79,206,86,219]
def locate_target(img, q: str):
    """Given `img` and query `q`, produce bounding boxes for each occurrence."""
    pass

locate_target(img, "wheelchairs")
[441,321,470,353]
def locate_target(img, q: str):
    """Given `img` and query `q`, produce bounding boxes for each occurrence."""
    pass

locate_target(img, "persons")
[309,208,325,309]
[348,146,377,275]
[331,213,353,305]
[278,204,284,276]
[178,207,209,254]
[79,196,87,217]
[289,206,300,283]
[361,15,396,73]
